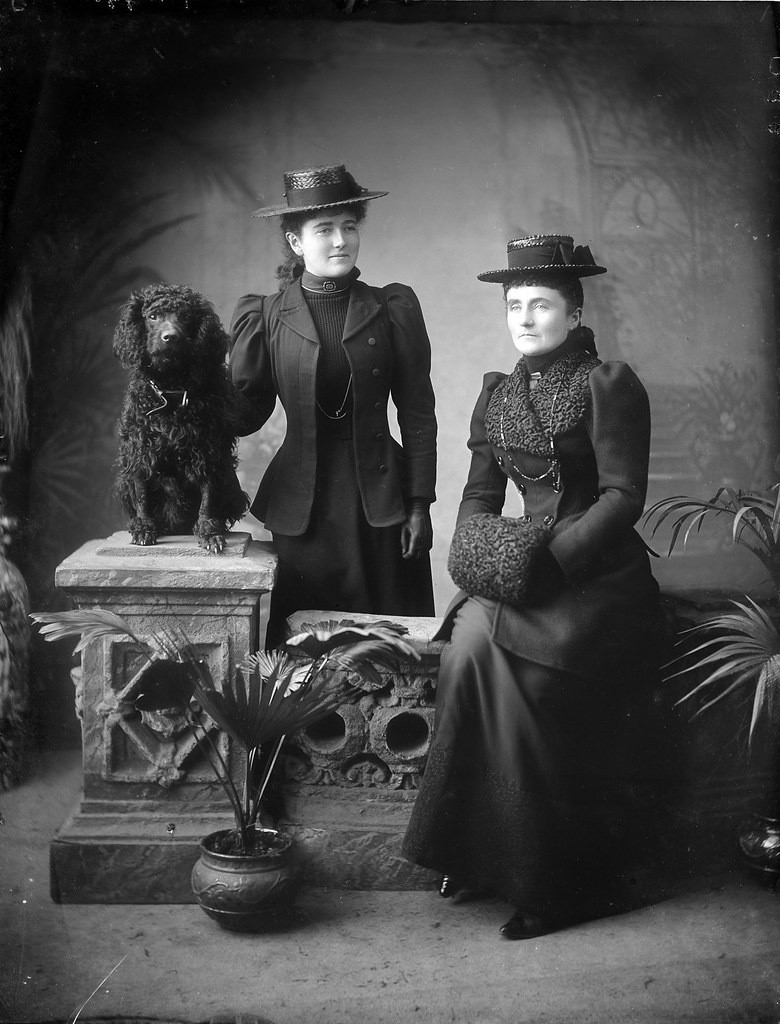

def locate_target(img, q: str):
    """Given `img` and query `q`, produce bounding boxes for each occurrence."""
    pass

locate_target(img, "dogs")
[113,287,252,554]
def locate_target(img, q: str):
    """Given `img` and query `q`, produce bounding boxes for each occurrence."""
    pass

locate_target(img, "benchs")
[282,608,720,890]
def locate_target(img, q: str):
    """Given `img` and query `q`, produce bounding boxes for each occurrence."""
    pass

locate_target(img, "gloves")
[401,498,433,561]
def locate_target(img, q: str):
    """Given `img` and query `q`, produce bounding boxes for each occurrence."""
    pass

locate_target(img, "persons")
[399,236,700,941]
[223,163,438,661]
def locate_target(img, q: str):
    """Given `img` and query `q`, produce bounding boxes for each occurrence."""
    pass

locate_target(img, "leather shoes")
[500,909,556,940]
[439,874,466,898]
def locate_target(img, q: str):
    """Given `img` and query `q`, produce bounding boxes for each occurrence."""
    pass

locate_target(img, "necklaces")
[500,354,568,482]
[318,368,351,419]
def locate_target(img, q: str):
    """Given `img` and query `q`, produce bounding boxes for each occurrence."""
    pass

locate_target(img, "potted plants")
[0,179,201,793]
[642,479,780,892]
[26,604,422,932]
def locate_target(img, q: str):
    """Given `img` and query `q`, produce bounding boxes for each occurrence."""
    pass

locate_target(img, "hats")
[251,164,390,218]
[477,234,607,283]
